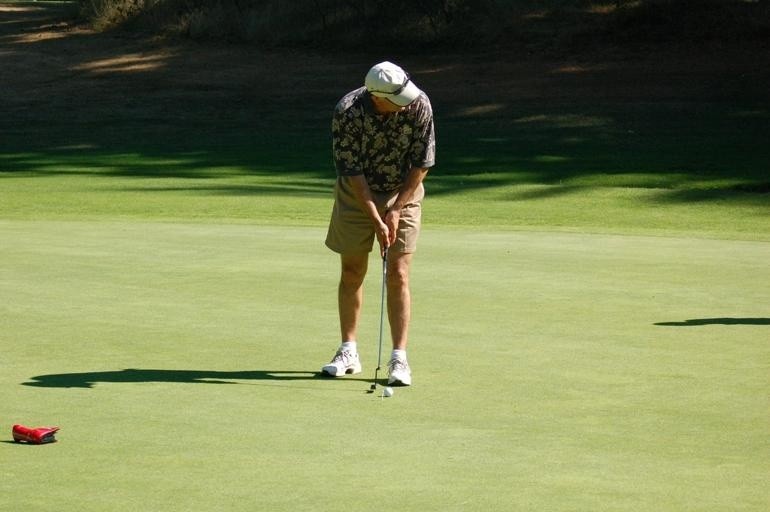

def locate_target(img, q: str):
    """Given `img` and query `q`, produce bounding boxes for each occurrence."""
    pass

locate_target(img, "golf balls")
[384,387,392,396]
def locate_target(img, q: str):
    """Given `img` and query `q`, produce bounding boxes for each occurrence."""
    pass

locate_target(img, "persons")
[320,61,436,387]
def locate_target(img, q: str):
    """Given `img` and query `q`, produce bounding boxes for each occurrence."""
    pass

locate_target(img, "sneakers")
[322,345,361,376]
[387,357,411,386]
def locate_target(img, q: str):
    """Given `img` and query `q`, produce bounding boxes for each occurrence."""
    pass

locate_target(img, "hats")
[365,61,421,108]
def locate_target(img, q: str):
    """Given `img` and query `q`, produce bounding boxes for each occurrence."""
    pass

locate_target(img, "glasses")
[367,71,410,96]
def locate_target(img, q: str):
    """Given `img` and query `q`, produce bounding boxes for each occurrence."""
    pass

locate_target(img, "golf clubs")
[370,242,388,394]
